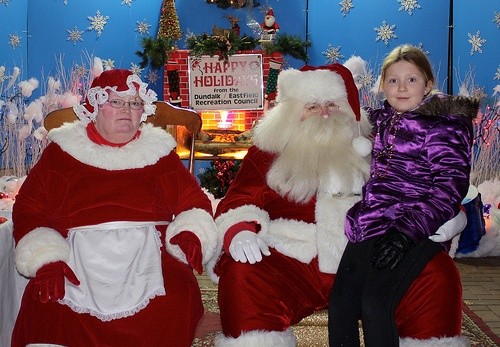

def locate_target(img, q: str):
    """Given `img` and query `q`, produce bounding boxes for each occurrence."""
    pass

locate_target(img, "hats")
[276,63,372,157]
[73,69,158,123]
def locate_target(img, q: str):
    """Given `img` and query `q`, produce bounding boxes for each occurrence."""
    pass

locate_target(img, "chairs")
[44,101,203,174]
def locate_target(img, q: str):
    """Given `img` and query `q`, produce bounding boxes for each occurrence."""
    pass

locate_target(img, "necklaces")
[375,112,400,178]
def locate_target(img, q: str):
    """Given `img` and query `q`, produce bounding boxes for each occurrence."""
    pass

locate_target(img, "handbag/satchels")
[458,193,486,254]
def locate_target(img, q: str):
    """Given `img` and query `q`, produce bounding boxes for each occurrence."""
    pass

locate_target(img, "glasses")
[305,105,340,112]
[106,99,144,109]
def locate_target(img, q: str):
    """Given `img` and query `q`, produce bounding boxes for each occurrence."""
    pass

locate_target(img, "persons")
[207,64,469,347]
[11,69,218,347]
[328,45,481,347]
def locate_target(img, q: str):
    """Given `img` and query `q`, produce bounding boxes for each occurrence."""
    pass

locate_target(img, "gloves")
[170,231,203,276]
[33,260,80,303]
[371,229,410,271]
[428,210,467,242]
[229,229,271,264]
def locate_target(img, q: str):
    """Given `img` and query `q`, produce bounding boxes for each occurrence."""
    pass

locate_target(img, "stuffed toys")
[258,10,280,34]
